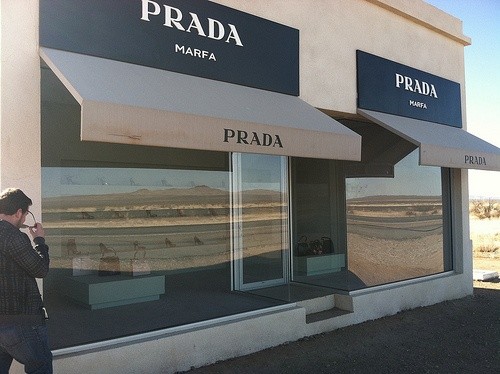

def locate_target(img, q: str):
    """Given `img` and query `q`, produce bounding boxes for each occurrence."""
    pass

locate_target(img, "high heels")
[99,243,112,253]
[67,239,82,255]
[165,238,176,247]
[194,236,205,245]
[134,241,146,250]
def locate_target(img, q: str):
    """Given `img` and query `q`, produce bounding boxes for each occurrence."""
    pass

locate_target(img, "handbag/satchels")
[71,249,92,276]
[298,235,334,257]
[129,248,150,277]
[99,247,120,277]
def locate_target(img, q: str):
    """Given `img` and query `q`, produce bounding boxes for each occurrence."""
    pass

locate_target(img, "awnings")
[40,60,420,178]
[357,107,500,171]
[39,46,361,162]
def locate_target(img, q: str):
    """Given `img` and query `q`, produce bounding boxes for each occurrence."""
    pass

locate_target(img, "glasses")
[20,211,37,229]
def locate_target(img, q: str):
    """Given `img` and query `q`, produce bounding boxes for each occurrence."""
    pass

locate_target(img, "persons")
[0,187,53,374]
